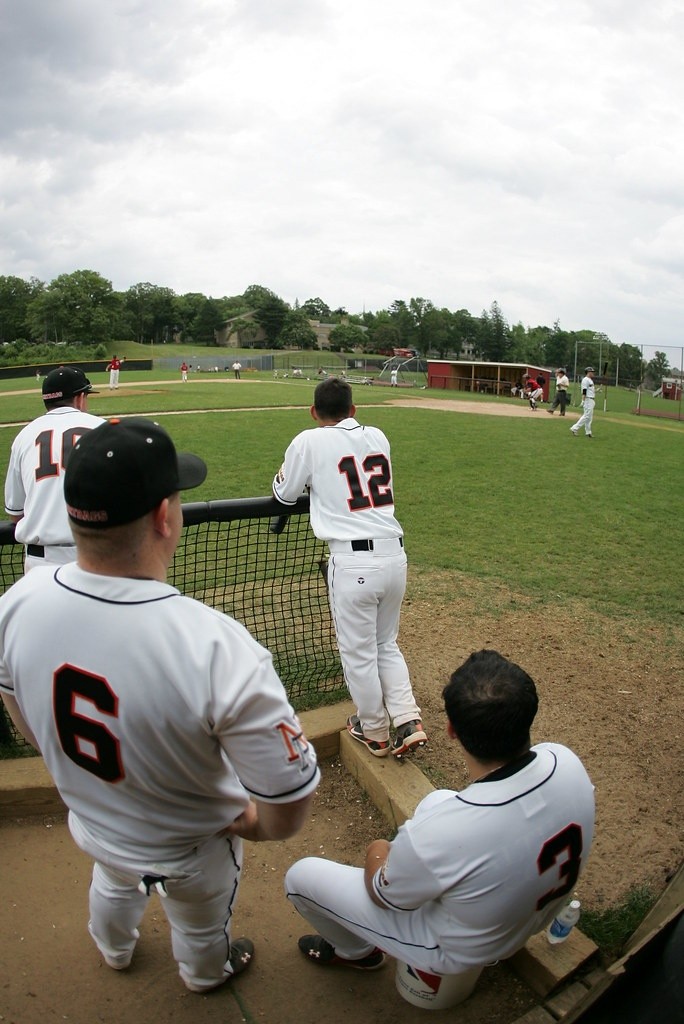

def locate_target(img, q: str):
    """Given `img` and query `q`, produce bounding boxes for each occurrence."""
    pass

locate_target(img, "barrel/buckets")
[395,958,484,1009]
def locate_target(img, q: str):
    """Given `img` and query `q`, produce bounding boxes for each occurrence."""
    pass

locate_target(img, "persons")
[232,361,241,379]
[0,416,321,993]
[272,377,428,759]
[36,369,40,381]
[285,649,596,977]
[570,367,596,438]
[292,366,346,377]
[547,369,570,415]
[181,363,188,382]
[106,354,126,390]
[391,366,397,387]
[515,373,546,411]
[5,365,107,575]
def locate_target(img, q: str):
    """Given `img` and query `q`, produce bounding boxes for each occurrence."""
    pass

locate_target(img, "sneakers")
[347,714,390,757]
[298,934,386,970]
[390,718,428,757]
[228,938,253,975]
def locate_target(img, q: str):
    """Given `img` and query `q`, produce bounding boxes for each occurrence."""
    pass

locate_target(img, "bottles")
[545,901,581,944]
[599,384,602,393]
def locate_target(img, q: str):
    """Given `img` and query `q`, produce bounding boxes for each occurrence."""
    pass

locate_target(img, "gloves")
[137,876,166,898]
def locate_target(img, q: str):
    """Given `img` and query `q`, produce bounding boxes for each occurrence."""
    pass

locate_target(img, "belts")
[557,389,566,392]
[28,544,44,557]
[352,537,404,551]
[586,396,595,400]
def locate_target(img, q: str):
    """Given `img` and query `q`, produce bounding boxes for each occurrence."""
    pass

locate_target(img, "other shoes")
[586,434,595,438]
[559,412,564,415]
[546,409,554,414]
[569,428,579,436]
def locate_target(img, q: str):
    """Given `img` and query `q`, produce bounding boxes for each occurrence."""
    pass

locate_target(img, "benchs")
[323,374,374,385]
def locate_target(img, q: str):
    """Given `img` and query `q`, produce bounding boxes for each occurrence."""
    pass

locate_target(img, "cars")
[46,342,83,346]
[1,341,16,345]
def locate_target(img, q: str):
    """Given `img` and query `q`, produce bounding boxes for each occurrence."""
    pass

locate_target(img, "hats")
[584,367,594,373]
[64,417,207,529]
[556,367,565,373]
[42,365,100,401]
[522,373,531,379]
